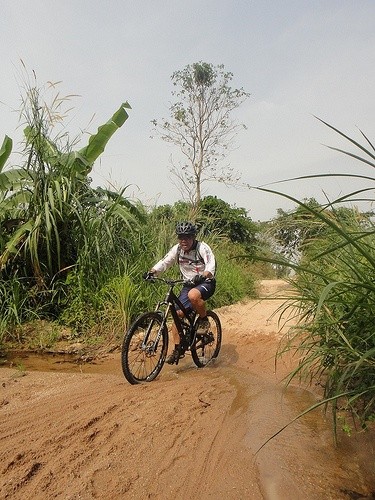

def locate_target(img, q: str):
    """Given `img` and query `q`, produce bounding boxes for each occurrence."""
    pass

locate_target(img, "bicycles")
[120,275,223,386]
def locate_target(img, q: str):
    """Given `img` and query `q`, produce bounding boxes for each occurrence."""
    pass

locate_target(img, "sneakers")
[165,349,183,363]
[196,319,211,335]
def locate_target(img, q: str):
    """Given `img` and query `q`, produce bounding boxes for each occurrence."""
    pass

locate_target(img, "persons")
[144,222,217,364]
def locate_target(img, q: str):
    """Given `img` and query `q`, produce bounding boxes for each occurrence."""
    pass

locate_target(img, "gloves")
[191,275,206,284]
[142,270,154,280]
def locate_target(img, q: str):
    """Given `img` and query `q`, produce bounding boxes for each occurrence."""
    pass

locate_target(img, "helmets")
[176,221,196,234]
